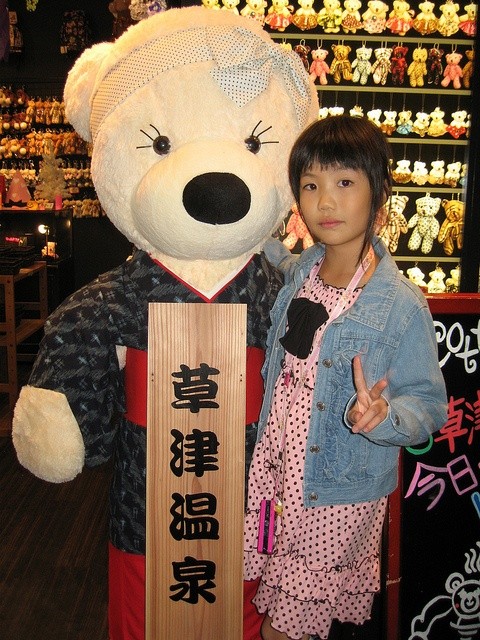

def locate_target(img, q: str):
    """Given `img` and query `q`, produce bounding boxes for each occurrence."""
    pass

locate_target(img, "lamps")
[37,223,59,259]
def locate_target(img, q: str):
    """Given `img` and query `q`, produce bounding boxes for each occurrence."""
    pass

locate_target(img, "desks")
[0,204,74,241]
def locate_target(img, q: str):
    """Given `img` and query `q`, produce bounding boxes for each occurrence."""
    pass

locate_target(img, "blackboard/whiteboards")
[386,294,480,640]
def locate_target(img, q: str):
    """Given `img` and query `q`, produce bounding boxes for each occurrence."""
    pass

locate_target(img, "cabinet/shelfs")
[164,0,480,295]
[0,81,108,218]
[1,260,49,437]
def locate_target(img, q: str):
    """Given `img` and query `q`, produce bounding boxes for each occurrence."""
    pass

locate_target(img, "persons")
[242,113,450,639]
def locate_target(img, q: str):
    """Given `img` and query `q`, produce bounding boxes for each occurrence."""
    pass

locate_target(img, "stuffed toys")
[240,0,268,31]
[459,163,467,187]
[339,1,363,34]
[390,45,408,85]
[0,85,106,216]
[396,110,413,135]
[265,1,295,32]
[10,4,320,635]
[459,1,478,38]
[425,48,444,86]
[461,48,475,88]
[380,194,409,254]
[292,44,310,72]
[406,47,428,88]
[392,159,412,184]
[366,109,382,128]
[406,197,441,255]
[445,265,461,294]
[447,110,467,138]
[351,46,373,85]
[318,0,343,34]
[410,160,428,186]
[437,0,460,37]
[427,266,447,293]
[441,51,463,89]
[386,1,415,37]
[290,0,319,31]
[362,1,389,35]
[329,43,354,84]
[380,111,398,136]
[282,201,315,250]
[427,107,448,137]
[428,159,444,185]
[411,111,430,138]
[405,266,427,287]
[308,48,331,85]
[437,198,465,254]
[370,47,393,85]
[444,161,461,187]
[412,1,438,36]
